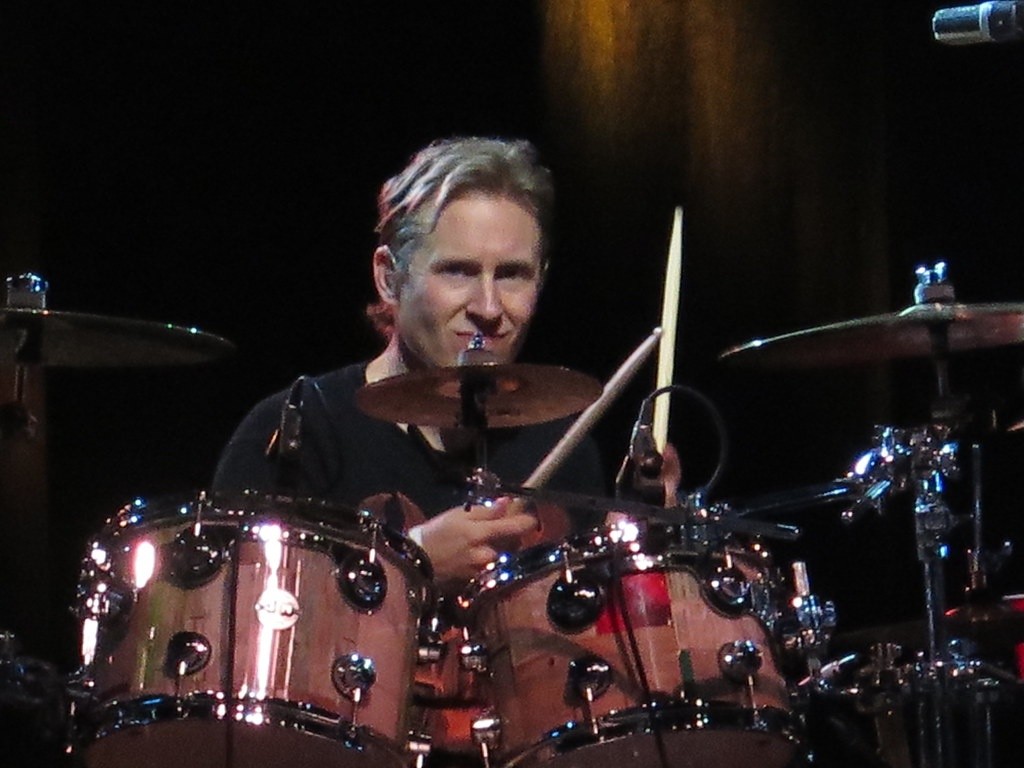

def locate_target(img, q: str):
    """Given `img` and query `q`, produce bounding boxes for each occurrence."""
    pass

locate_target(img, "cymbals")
[718,301,1024,374]
[0,302,232,369]
[354,363,606,428]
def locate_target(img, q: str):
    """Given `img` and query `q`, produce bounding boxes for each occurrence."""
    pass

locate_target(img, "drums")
[469,526,796,768]
[65,486,434,766]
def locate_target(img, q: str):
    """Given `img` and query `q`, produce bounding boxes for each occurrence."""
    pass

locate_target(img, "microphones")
[614,397,656,500]
[269,376,310,488]
[931,0,1024,46]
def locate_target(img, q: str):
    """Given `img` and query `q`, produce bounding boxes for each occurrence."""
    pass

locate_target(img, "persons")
[208,138,682,760]
